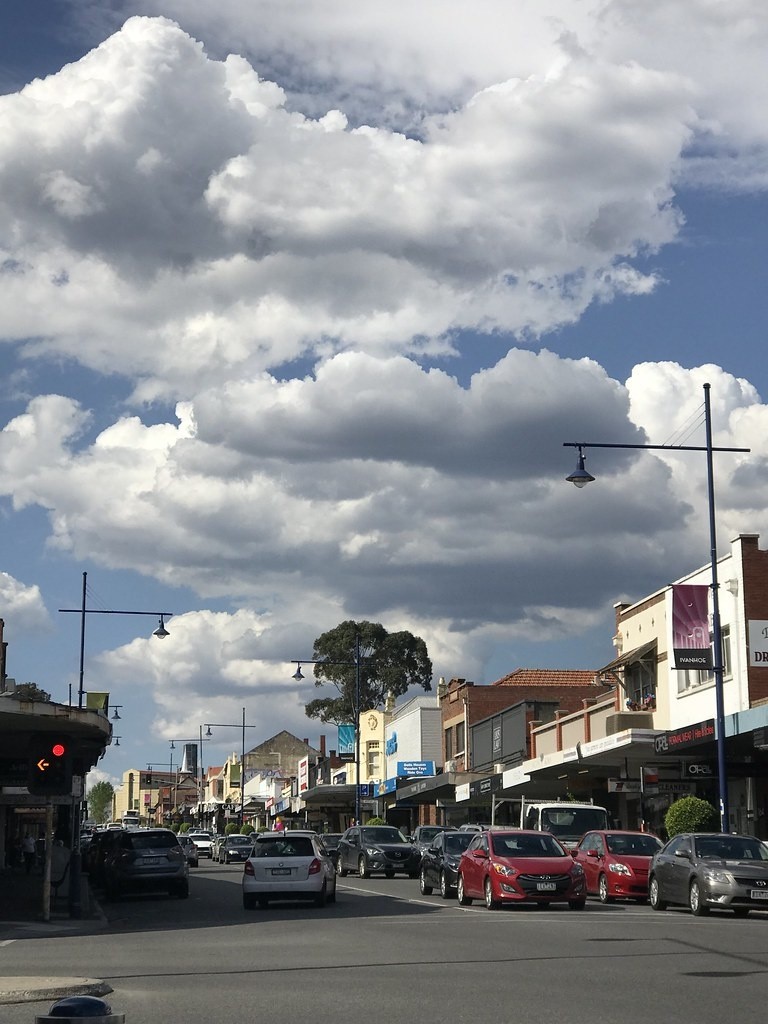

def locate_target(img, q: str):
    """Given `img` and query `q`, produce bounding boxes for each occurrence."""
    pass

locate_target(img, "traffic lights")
[27,731,72,796]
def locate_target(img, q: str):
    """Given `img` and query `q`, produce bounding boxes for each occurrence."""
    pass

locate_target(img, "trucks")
[488,794,613,853]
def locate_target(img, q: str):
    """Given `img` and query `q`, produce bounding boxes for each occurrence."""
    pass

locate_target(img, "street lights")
[69,682,123,721]
[563,384,750,834]
[291,634,377,825]
[205,708,256,827]
[146,725,210,828]
[58,572,174,710]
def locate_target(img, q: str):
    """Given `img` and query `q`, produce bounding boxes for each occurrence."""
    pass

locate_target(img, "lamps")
[644,693,655,706]
[626,698,641,712]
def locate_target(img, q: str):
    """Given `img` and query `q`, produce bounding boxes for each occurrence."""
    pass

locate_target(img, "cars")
[81,817,189,902]
[242,835,336,910]
[412,823,492,855]
[336,826,420,879]
[570,830,665,904]
[420,831,482,899]
[457,830,587,910]
[648,833,768,916]
[177,827,344,867]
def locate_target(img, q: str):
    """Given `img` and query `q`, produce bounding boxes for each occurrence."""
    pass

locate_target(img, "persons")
[533,812,555,836]
[4,829,21,872]
[20,833,37,873]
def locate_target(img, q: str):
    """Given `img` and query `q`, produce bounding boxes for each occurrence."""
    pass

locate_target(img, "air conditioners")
[445,761,458,773]
[493,764,505,774]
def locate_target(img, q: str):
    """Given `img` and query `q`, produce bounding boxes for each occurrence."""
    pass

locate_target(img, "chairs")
[51,862,70,900]
